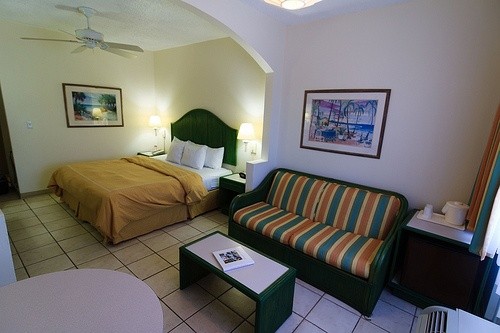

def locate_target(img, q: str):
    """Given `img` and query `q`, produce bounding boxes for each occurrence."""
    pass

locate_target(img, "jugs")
[442,200,470,226]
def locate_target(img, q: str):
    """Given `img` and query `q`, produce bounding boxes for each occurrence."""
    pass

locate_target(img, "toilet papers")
[444,200,468,226]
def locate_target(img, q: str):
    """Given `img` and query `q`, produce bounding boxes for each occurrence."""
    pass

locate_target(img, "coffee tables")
[179,230,296,333]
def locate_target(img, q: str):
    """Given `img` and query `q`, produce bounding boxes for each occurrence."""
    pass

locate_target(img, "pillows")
[204,147,224,169]
[166,136,186,164]
[181,140,207,170]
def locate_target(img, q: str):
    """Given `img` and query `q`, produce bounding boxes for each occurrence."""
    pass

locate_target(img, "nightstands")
[137,149,164,157]
[219,172,246,216]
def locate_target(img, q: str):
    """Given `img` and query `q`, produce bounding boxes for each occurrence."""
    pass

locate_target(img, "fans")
[19,6,144,59]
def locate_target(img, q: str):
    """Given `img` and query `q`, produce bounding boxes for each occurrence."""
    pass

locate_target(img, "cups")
[423,204,433,219]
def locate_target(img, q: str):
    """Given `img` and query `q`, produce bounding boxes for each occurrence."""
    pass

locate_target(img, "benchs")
[228,168,409,321]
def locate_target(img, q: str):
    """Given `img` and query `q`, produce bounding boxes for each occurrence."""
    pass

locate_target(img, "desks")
[0,269,164,333]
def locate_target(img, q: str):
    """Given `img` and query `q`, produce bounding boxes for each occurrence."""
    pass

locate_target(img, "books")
[212,247,255,272]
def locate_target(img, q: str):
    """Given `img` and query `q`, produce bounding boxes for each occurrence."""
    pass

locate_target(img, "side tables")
[386,208,493,316]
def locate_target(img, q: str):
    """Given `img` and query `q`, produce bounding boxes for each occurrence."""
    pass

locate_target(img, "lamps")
[263,0,323,11]
[237,123,256,153]
[149,116,161,154]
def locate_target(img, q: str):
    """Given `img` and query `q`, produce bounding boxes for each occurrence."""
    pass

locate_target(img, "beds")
[47,154,233,245]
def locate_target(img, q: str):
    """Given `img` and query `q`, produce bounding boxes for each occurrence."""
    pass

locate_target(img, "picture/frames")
[62,83,124,128]
[300,88,391,159]
